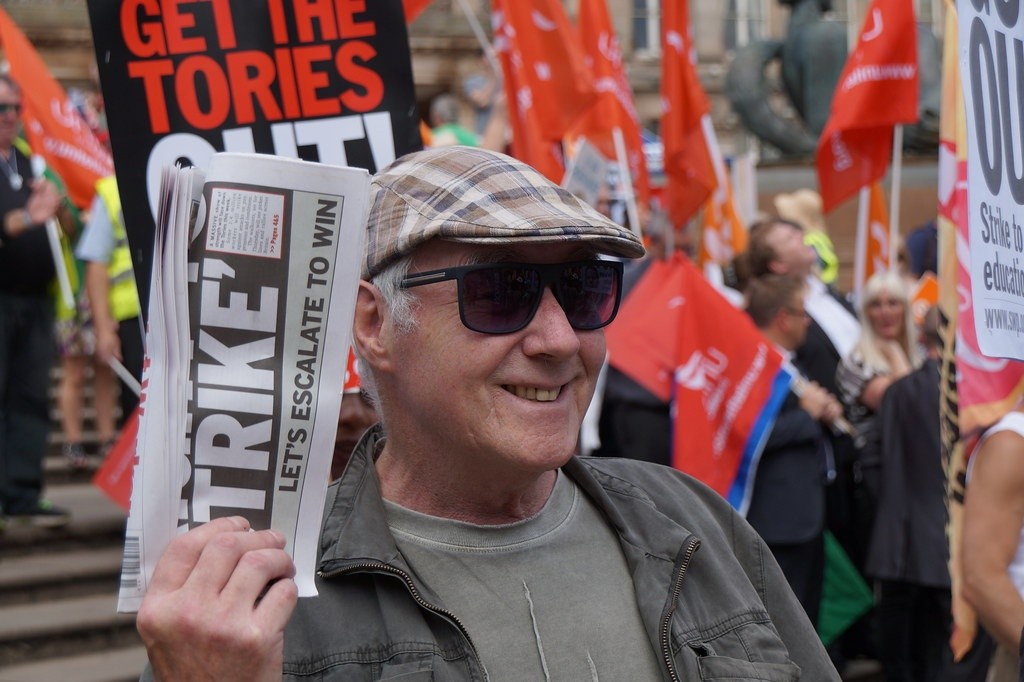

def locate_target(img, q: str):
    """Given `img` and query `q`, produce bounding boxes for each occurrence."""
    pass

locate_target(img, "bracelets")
[19,208,33,229]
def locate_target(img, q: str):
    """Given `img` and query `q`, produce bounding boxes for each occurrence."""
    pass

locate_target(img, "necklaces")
[0,148,23,189]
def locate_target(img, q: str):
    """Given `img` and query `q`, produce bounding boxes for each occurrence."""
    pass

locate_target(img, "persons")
[0,72,1024,682]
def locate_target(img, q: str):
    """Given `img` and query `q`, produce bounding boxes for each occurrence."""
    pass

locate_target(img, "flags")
[814,0,919,213]
[866,187,890,282]
[660,1,719,230]
[0,5,114,210]
[579,0,653,210]
[489,0,597,187]
[936,0,1024,660]
[602,248,799,517]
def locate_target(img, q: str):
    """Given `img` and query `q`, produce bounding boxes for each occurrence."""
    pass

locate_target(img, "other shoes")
[62,444,86,471]
[98,439,116,457]
[0,503,71,529]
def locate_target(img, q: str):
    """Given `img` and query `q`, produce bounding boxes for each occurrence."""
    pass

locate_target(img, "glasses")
[0,103,20,113]
[394,260,624,335]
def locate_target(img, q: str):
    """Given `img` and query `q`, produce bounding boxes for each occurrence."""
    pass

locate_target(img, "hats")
[361,145,647,292]
